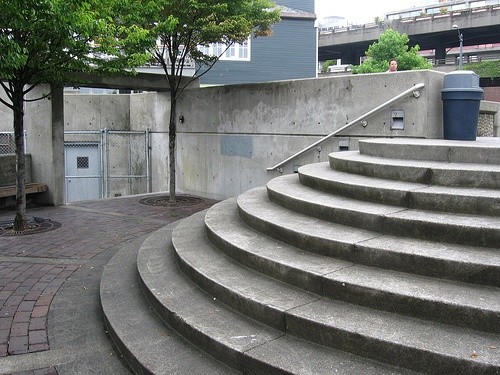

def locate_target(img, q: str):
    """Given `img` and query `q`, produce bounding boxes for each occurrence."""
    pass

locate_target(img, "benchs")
[0,152,48,198]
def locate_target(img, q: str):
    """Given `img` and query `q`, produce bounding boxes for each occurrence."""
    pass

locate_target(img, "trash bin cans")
[440,71,485,141]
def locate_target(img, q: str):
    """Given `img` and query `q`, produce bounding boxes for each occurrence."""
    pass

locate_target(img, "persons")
[385,59,399,72]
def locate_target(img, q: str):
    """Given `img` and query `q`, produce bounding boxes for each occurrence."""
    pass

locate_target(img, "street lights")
[452,24,464,70]
